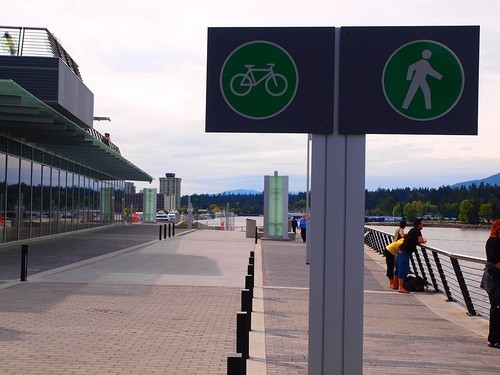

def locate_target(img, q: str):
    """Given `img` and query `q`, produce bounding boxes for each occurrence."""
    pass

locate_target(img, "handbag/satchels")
[403,275,424,292]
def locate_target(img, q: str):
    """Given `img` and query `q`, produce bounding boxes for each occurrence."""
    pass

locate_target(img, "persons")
[385,234,409,288]
[393,220,408,242]
[485,219,500,348]
[291,216,298,234]
[390,219,427,293]
[103,133,110,147]
[297,213,306,242]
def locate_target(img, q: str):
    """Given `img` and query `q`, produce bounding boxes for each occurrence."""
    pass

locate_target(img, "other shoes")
[487,341,500,348]
